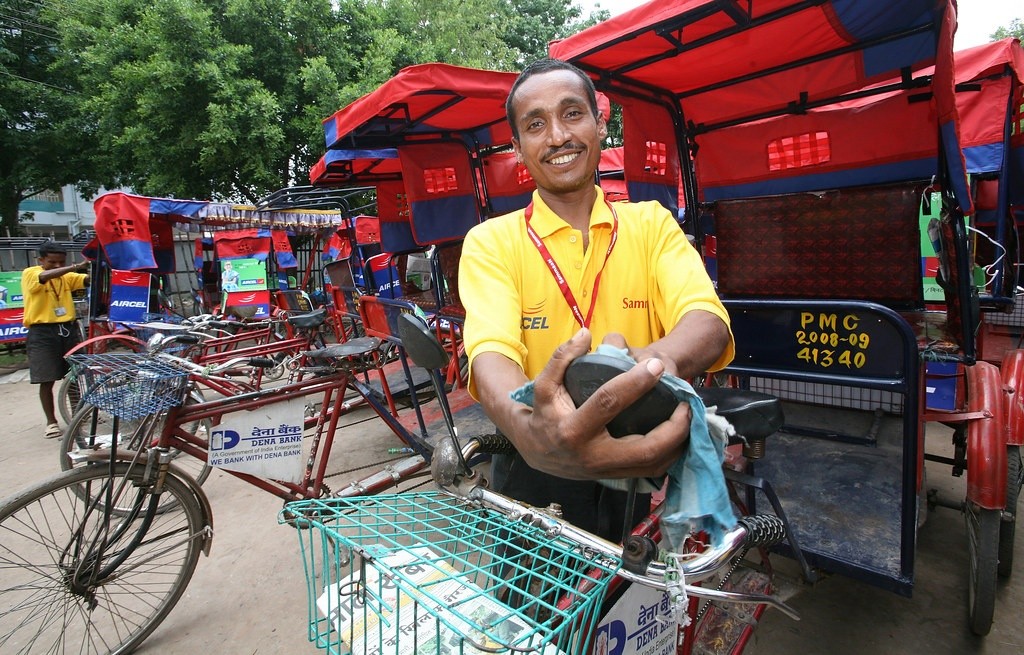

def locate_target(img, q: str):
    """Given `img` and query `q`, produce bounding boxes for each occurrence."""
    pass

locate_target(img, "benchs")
[712,181,977,366]
[388,237,465,326]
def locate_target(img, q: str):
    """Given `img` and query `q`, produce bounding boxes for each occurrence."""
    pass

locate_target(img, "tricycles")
[0,0,1024,655]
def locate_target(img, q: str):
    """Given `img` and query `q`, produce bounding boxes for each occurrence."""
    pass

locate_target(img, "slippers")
[44,423,62,439]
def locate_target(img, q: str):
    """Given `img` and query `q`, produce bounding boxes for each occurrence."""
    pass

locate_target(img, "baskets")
[65,352,191,420]
[277,489,623,655]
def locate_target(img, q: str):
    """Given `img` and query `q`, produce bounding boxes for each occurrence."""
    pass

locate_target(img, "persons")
[20,243,91,437]
[457,60,735,621]
[222,262,239,292]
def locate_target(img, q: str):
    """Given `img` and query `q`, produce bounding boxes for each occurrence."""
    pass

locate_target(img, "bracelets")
[73,264,77,273]
